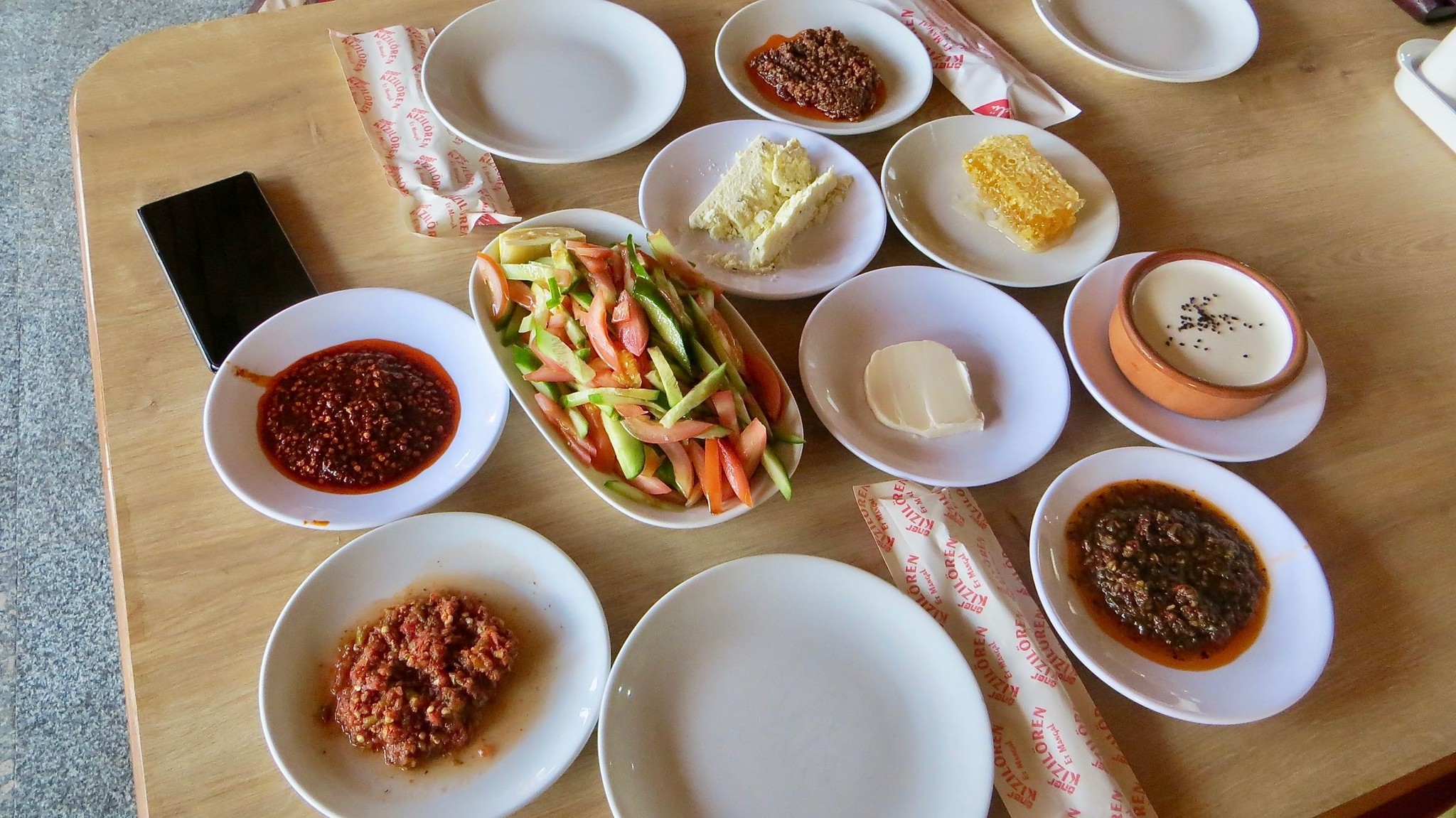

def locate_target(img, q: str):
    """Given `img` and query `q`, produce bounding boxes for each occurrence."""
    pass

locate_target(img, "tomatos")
[478,230,781,514]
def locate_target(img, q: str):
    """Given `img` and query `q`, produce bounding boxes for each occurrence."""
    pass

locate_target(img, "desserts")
[962,133,1088,249]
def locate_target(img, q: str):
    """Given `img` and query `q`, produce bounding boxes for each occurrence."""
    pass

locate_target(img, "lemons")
[499,227,589,265]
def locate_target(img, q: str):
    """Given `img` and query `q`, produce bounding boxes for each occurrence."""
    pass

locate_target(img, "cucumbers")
[499,234,810,504]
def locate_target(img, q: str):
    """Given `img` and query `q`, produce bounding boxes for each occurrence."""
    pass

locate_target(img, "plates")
[1029,447,1336,725]
[257,510,611,818]
[201,287,511,533]
[468,208,805,531]
[638,120,888,302]
[1029,0,1261,85]
[1062,251,1328,462]
[880,113,1121,289]
[598,552,995,818]
[797,265,1072,488]
[1394,37,1456,155]
[714,0,933,135]
[421,1,687,164]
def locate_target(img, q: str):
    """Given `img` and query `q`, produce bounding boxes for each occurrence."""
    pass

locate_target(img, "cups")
[1417,27,1456,111]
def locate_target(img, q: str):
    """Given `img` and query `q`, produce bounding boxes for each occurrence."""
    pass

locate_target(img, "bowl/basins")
[1108,247,1309,421]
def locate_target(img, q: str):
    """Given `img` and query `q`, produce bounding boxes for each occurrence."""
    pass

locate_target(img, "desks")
[69,0,1456,818]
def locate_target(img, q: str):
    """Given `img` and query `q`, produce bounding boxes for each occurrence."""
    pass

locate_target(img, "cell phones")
[135,170,320,373]
